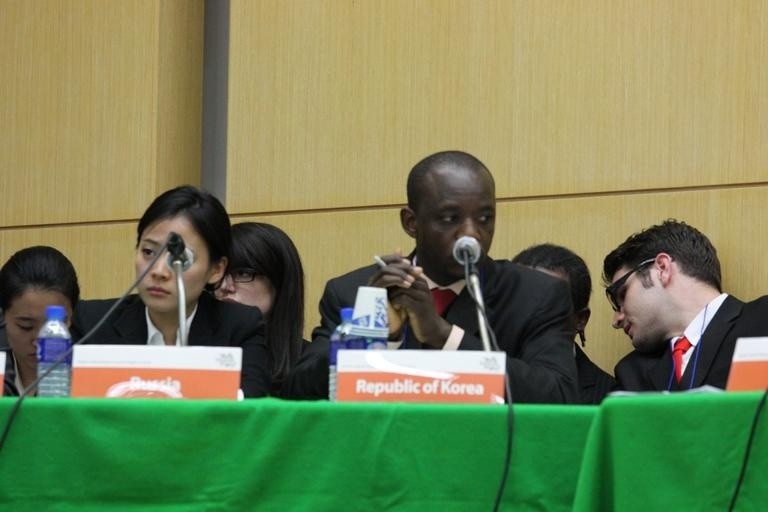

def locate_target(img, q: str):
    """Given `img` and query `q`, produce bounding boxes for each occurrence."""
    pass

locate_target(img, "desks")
[0,388,768,512]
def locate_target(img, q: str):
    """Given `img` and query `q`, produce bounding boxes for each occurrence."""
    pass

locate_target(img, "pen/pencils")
[373,255,387,267]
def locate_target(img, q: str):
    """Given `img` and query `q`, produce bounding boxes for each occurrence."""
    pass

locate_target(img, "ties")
[672,338,693,382]
[430,288,457,315]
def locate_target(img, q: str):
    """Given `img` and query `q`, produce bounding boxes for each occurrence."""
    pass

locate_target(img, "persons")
[209,222,320,401]
[0,246,98,398]
[290,150,579,405]
[68,185,287,401]
[510,244,614,405]
[601,218,764,392]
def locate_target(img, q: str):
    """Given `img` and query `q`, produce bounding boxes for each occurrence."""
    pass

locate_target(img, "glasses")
[606,256,675,311]
[228,267,265,283]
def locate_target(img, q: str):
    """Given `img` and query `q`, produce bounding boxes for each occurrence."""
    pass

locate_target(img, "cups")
[350,286,390,339]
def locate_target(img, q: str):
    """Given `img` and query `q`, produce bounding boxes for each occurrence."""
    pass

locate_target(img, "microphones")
[452,235,491,351]
[165,232,194,346]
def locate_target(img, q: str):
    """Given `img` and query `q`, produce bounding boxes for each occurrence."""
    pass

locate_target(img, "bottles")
[329,308,367,401]
[37,306,72,396]
